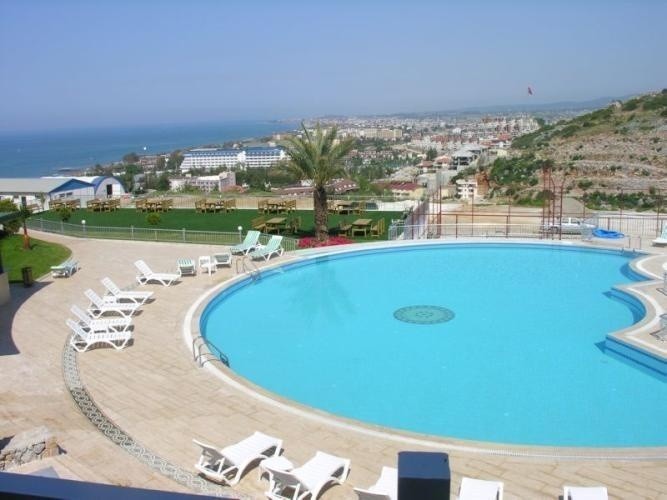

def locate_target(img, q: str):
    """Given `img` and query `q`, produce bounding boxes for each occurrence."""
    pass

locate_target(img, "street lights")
[81,219,86,233]
[237,225,243,244]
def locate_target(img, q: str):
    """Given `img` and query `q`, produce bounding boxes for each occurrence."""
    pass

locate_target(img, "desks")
[258,456,293,485]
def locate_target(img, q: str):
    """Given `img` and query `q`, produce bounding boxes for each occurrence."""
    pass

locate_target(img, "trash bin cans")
[21,266,33,286]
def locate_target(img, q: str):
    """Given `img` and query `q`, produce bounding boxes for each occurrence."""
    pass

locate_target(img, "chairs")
[65,276,153,353]
[175,230,284,275]
[354,466,400,500]
[458,475,503,500]
[266,451,351,500]
[191,430,284,485]
[134,259,180,287]
[563,484,608,500]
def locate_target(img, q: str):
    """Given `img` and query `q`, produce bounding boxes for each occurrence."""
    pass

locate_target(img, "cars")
[546,217,595,234]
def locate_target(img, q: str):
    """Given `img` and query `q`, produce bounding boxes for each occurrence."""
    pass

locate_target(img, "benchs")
[251,198,302,234]
[193,199,237,216]
[48,198,81,211]
[326,199,385,240]
[134,199,173,213]
[85,199,121,213]
